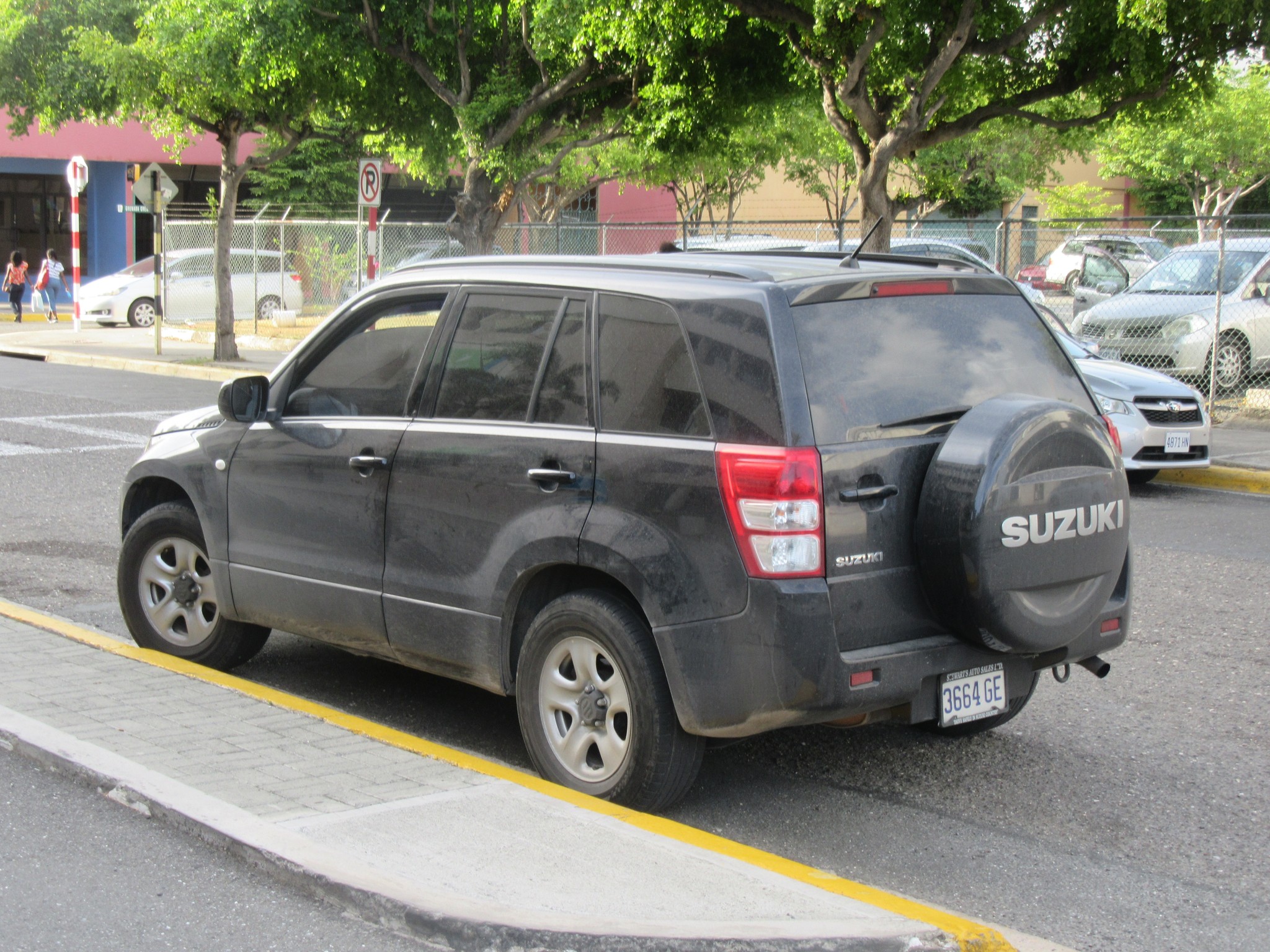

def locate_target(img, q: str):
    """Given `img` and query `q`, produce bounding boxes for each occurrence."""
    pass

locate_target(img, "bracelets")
[30,285,33,288]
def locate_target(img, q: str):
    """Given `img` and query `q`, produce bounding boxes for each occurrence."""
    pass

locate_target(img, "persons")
[34,248,70,323]
[2,250,34,323]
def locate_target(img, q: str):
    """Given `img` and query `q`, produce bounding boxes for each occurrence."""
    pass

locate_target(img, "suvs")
[341,241,507,302]
[114,239,1134,816]
[651,232,1043,304]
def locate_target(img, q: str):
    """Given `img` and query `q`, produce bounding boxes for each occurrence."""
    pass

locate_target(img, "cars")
[1013,249,1068,291]
[1036,303,1212,484]
[1067,234,1270,394]
[78,248,304,328]
[1045,234,1202,297]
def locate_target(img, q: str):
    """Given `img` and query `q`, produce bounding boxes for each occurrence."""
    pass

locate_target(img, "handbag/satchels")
[37,259,49,290]
[31,290,44,312]
[5,286,9,292]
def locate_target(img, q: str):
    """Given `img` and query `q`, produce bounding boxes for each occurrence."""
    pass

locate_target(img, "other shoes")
[14,317,21,322]
[50,317,58,323]
[45,313,51,322]
